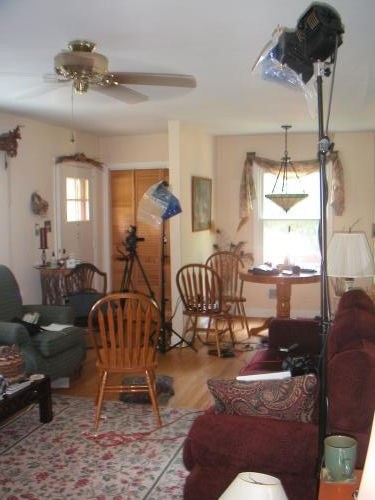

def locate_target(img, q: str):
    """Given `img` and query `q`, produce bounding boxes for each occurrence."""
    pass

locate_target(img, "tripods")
[118,245,157,310]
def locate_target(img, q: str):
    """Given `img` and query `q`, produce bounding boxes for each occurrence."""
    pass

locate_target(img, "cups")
[66,259,76,269]
[57,259,65,269]
[323,435,358,479]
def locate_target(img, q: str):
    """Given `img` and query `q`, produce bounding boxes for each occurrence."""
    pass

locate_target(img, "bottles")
[61,248,67,268]
[51,250,56,269]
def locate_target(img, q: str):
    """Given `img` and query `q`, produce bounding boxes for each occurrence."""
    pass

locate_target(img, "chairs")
[175,252,250,359]
[64,264,162,429]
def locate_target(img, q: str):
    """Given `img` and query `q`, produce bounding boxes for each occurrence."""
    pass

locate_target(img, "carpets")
[0,393,201,500]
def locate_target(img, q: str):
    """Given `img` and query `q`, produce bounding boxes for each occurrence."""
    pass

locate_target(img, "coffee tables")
[0,372,53,425]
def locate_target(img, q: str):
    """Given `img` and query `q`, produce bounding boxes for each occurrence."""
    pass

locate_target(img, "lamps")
[218,472,289,500]
[326,232,375,293]
[265,124,309,214]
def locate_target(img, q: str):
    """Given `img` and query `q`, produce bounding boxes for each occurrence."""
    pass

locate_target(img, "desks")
[36,261,84,304]
[239,268,321,336]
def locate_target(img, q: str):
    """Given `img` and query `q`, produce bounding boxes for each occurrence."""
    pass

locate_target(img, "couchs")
[182,289,375,500]
[0,266,86,384]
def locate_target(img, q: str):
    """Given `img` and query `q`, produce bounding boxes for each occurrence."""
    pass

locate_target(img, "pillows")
[207,374,317,423]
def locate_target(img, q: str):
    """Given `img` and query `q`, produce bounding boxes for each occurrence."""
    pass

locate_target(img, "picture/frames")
[192,176,213,231]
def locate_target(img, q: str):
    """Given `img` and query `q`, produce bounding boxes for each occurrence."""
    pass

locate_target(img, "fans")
[0,38,197,105]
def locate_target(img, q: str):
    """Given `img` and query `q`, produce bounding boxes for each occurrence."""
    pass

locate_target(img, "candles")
[40,227,47,248]
